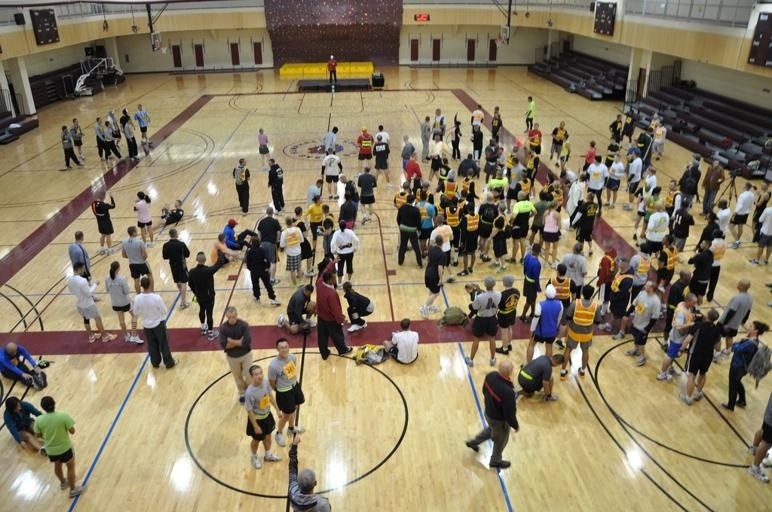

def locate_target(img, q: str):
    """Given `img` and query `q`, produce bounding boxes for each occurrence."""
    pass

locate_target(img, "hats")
[545,284,556,299]
[228,219,238,225]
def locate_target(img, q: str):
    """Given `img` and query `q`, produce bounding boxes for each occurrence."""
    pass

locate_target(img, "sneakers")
[61,478,69,490]
[419,305,429,319]
[124,333,131,342]
[278,313,285,328]
[208,331,219,341]
[560,370,568,381]
[39,447,47,456]
[656,371,669,381]
[764,259,768,265]
[468,267,473,274]
[612,333,625,340]
[747,259,758,266]
[324,349,330,360]
[271,299,281,305]
[102,333,118,343]
[287,427,304,435]
[735,401,746,407]
[748,445,769,458]
[490,461,511,468]
[712,352,723,364]
[38,371,47,388]
[668,367,680,377]
[251,456,262,468]
[457,271,468,276]
[129,335,144,343]
[467,440,479,452]
[88,333,101,343]
[201,324,208,335]
[465,357,474,366]
[507,344,513,350]
[429,305,439,312]
[578,367,585,380]
[625,349,640,356]
[180,303,189,309]
[490,357,496,366]
[339,347,352,355]
[598,323,613,333]
[720,401,734,412]
[275,431,285,446]
[356,322,367,330]
[32,375,42,391]
[554,340,565,350]
[694,391,703,401]
[678,392,693,405]
[721,348,732,358]
[496,348,508,355]
[347,324,358,332]
[748,465,769,483]
[488,263,500,268]
[496,267,508,273]
[69,486,86,497]
[265,454,282,462]
[637,356,646,366]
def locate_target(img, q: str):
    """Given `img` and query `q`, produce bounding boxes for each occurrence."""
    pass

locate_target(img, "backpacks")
[483,207,495,223]
[740,338,772,385]
[439,304,469,331]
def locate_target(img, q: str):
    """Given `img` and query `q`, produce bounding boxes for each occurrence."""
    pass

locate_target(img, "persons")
[457,153,481,180]
[404,152,422,182]
[338,192,357,229]
[70,117,88,159]
[653,235,678,320]
[560,243,587,299]
[752,179,771,243]
[292,206,317,276]
[560,285,602,381]
[327,54,338,83]
[66,262,119,343]
[509,157,525,191]
[306,180,322,208]
[478,192,498,262]
[572,192,597,256]
[425,129,445,182]
[686,154,701,173]
[749,200,772,265]
[122,225,154,294]
[496,145,507,166]
[623,148,643,212]
[34,395,88,494]
[545,194,556,210]
[91,186,116,259]
[596,247,618,324]
[68,231,92,286]
[436,193,448,215]
[605,137,619,169]
[258,127,274,164]
[417,181,434,200]
[699,158,725,220]
[339,174,360,203]
[133,276,180,370]
[598,257,634,340]
[322,126,339,155]
[505,191,538,264]
[232,158,250,213]
[223,218,258,252]
[530,190,544,246]
[427,215,454,278]
[526,284,562,363]
[582,140,597,176]
[522,96,536,132]
[267,338,305,445]
[625,367,626,367]
[469,103,484,127]
[145,200,184,233]
[542,172,554,193]
[494,275,520,355]
[484,169,509,190]
[106,110,124,150]
[279,216,305,288]
[331,219,358,282]
[444,200,464,249]
[375,125,390,144]
[279,438,334,512]
[604,154,625,210]
[330,219,360,289]
[701,238,714,264]
[95,118,113,161]
[134,103,152,144]
[434,158,451,195]
[649,250,669,293]
[414,194,435,257]
[394,195,423,271]
[549,122,567,168]
[654,120,667,161]
[489,111,501,140]
[356,127,374,176]
[663,270,692,352]
[340,282,377,331]
[682,309,718,406]
[1,342,47,391]
[317,205,336,257]
[452,195,458,205]
[471,123,483,161]
[59,126,83,169]
[435,169,459,196]
[636,128,646,150]
[509,145,524,159]
[728,183,757,249]
[266,159,288,216]
[644,197,670,255]
[373,135,393,188]
[747,391,772,482]
[257,208,284,284]
[713,279,753,362]
[392,181,415,210]
[120,107,136,135]
[542,202,563,268]
[551,180,564,212]
[720,321,769,411]
[568,174,589,230]
[492,186,505,204]
[419,235,445,319]
[400,135,415,171]
[609,114,623,150]
[513,168,532,201]
[658,295,702,380]
[484,139,501,182]
[219,307,261,403]
[688,250,713,304]
[630,243,650,298]
[104,261,142,342]
[342,281,374,332]
[188,252,226,342]
[461,167,480,200]
[465,359,519,468]
[633,181,654,240]
[528,123,543,157]
[450,112,463,162]
[492,106,501,116]
[637,186,665,246]
[459,189,468,197]
[692,212,721,254]
[544,263,576,350]
[626,281,661,366]
[664,180,681,234]
[382,318,421,364]
[624,138,638,193]
[622,110,636,150]
[603,138,619,167]
[134,192,158,248]
[315,256,353,360]
[672,200,695,252]
[718,200,731,239]
[637,126,655,167]
[463,283,488,319]
[604,135,620,169]
[644,166,657,194]
[684,171,699,198]
[649,112,662,136]
[210,235,248,265]
[487,203,508,275]
[357,166,377,224]
[239,364,285,468]
[707,229,726,302]
[277,284,315,336]
[459,197,463,207]
[677,160,702,211]
[420,116,433,163]
[457,202,481,277]
[586,156,609,218]
[465,277,496,367]
[3,396,48,458]
[124,115,140,159]
[514,354,564,401]
[432,109,446,142]
[245,237,281,307]
[321,147,342,199]
[559,132,571,174]
[302,196,325,251]
[560,166,579,188]
[161,228,196,311]
[102,120,125,160]
